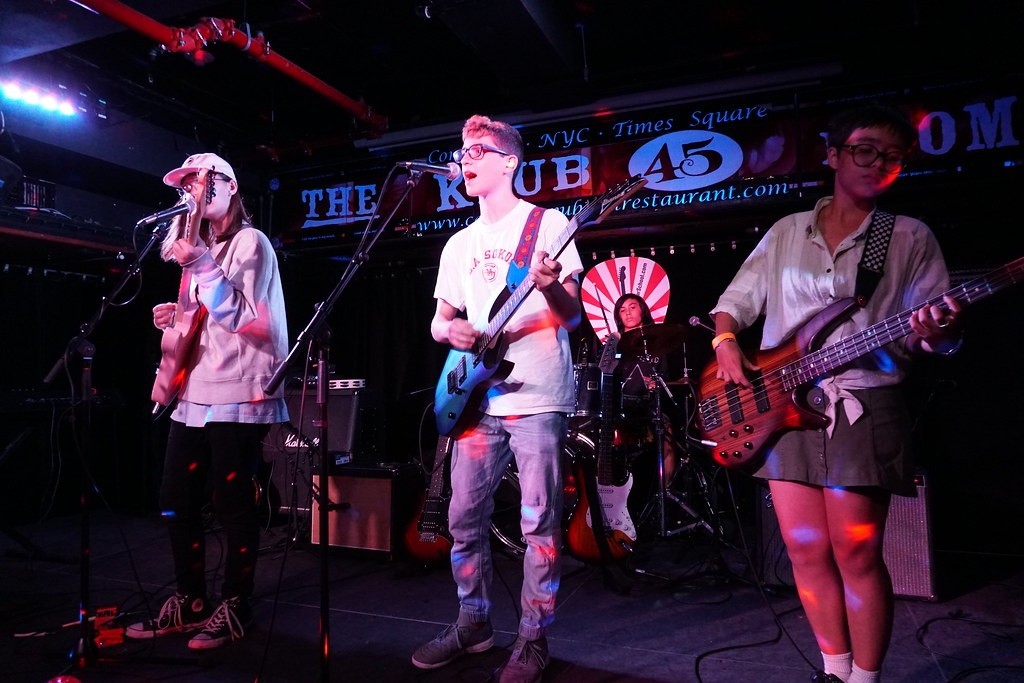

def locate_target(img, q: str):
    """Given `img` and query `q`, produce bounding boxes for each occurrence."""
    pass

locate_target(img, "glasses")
[451,144,508,163]
[175,178,228,192]
[833,140,908,175]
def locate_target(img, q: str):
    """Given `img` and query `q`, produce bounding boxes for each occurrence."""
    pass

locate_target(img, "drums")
[488,429,595,556]
[567,363,625,434]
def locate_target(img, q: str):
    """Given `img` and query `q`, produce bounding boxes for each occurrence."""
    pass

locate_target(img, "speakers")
[757,471,944,602]
[260,388,420,562]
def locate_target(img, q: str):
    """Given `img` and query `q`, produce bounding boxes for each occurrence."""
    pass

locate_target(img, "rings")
[936,321,949,328]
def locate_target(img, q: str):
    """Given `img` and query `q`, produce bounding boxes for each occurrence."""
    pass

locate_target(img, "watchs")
[938,335,963,356]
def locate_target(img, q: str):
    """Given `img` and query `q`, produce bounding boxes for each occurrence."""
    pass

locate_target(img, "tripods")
[638,330,739,551]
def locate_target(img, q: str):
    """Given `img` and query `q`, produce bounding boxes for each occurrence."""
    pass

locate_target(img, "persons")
[604,293,673,490]
[411,115,585,683]
[126,153,289,649]
[707,107,962,683]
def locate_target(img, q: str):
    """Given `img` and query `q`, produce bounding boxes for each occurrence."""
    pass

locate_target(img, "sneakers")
[411,611,496,669]
[186,597,255,650]
[124,593,212,639]
[498,633,550,683]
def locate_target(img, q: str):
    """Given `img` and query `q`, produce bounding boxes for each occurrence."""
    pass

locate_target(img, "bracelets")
[711,333,735,351]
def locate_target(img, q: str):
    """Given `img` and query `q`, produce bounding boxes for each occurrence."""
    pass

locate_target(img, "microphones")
[397,161,462,180]
[137,198,198,227]
[585,348,593,355]
[689,316,716,331]
[332,502,351,513]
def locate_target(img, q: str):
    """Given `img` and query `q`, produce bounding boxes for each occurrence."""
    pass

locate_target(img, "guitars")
[696,256,1024,469]
[401,436,454,567]
[432,173,649,440]
[563,332,639,565]
[149,165,216,423]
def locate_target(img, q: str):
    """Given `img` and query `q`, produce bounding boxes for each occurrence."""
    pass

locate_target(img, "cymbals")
[665,378,695,388]
[616,320,693,360]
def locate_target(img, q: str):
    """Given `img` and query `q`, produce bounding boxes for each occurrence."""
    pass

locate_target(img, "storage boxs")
[307,463,423,562]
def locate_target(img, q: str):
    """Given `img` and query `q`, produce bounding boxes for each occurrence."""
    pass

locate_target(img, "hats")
[163,153,237,187]
